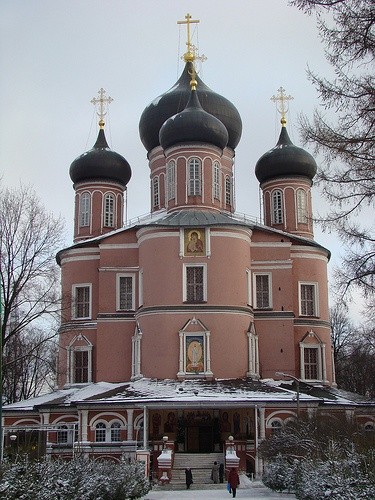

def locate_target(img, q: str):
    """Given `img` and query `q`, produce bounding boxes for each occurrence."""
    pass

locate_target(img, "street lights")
[274,371,301,420]
[9,434,17,462]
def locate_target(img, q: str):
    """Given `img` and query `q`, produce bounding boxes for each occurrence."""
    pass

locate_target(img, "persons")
[185,467,193,490]
[211,461,219,484]
[227,467,241,497]
[218,463,225,484]
